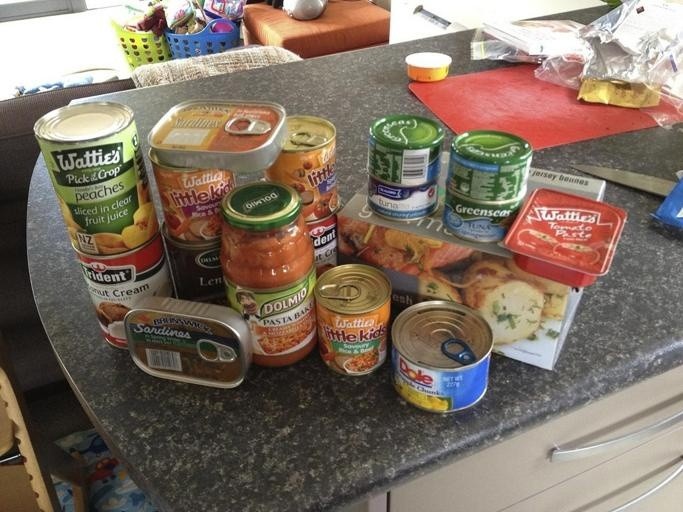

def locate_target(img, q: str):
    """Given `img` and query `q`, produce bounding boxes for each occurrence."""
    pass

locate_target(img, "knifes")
[570,162,678,197]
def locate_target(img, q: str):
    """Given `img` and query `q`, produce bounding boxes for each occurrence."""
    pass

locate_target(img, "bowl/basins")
[405,52,452,82]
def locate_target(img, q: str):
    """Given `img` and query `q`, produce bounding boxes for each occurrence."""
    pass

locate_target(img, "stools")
[242,3,391,57]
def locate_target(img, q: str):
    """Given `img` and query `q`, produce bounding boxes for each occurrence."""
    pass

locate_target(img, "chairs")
[1,72,132,392]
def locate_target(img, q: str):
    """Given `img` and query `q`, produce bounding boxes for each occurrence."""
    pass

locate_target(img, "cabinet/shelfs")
[388,361,682,511]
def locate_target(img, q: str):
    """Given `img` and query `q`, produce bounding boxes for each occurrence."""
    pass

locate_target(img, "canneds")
[161,219,222,306]
[221,181,318,368]
[445,130,532,203]
[368,175,439,221]
[444,186,522,244]
[264,115,339,225]
[72,233,178,354]
[304,195,339,276]
[123,298,253,390]
[148,100,288,176]
[391,300,494,415]
[313,263,392,379]
[367,114,444,187]
[33,100,159,255]
[147,147,236,244]
